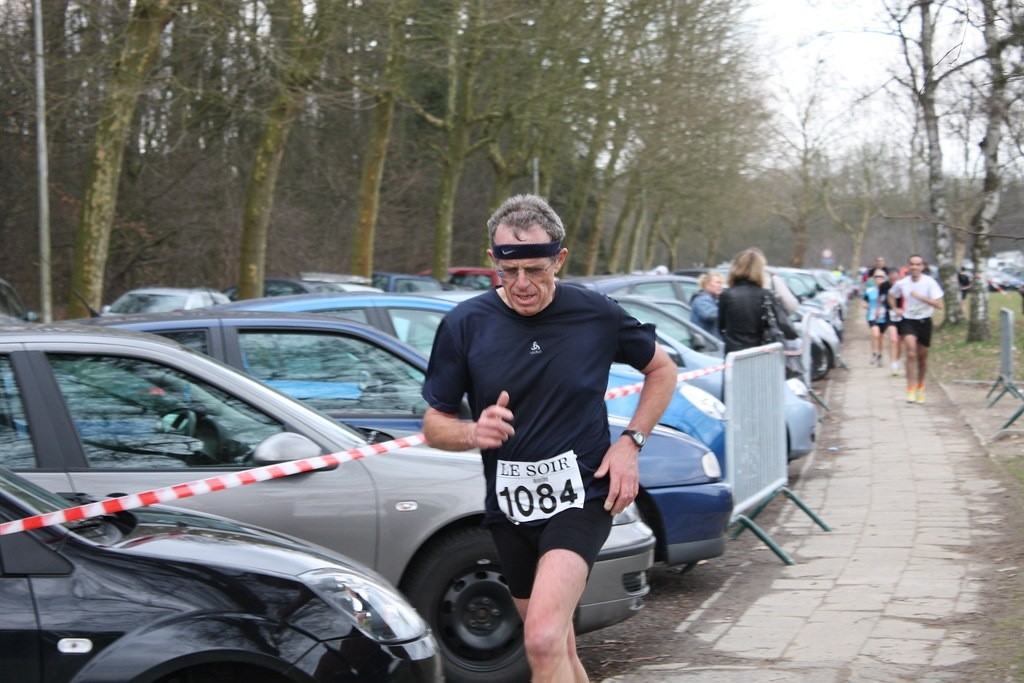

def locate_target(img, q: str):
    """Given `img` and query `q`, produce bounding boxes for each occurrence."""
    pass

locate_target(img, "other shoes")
[916,385,924,404]
[906,386,916,403]
[892,362,905,377]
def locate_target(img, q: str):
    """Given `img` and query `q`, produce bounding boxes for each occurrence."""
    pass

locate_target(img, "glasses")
[493,259,557,278]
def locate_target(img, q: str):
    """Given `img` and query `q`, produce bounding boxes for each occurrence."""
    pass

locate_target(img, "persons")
[419,194,677,683]
[886,254,945,404]
[719,247,799,354]
[861,257,969,375]
[688,273,722,343]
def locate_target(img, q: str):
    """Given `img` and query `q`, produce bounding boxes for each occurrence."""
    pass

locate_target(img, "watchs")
[622,429,645,452]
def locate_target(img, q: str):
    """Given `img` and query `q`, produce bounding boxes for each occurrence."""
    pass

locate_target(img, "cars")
[927,247,1023,294]
[80,312,734,569]
[0,465,445,683]
[96,287,232,320]
[257,265,858,463]
[121,292,742,506]
[0,323,657,683]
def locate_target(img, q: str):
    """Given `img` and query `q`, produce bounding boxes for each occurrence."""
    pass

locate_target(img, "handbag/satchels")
[762,292,785,345]
